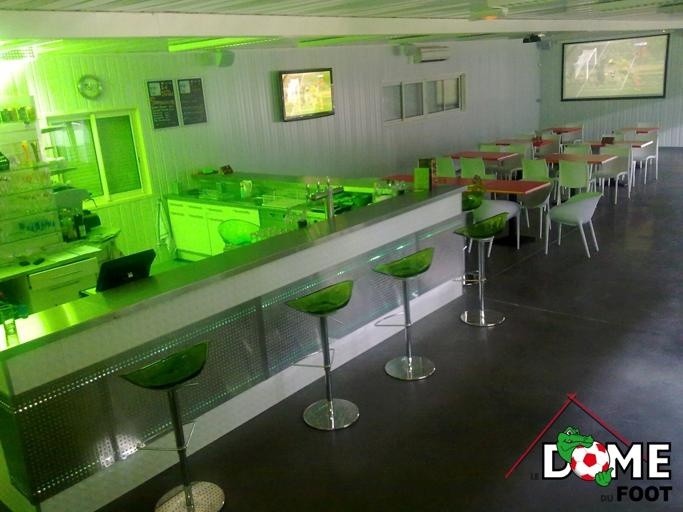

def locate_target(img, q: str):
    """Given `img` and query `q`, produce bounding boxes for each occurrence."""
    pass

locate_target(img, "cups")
[372,179,406,197]
[0,303,15,332]
[5,331,19,346]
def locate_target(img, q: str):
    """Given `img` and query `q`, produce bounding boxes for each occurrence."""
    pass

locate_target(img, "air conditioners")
[414,43,452,63]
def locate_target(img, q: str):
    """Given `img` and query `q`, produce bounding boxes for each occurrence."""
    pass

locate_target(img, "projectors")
[522,34,542,44]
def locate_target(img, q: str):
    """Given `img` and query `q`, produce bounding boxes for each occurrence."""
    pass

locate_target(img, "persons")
[604,40,656,91]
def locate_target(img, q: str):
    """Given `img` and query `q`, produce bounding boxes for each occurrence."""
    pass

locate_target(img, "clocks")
[77,74,103,101]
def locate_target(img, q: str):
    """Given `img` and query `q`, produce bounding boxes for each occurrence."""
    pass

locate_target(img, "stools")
[454,207,510,329]
[373,244,438,381]
[220,216,260,252]
[463,186,484,288]
[119,340,232,512]
[285,280,363,428]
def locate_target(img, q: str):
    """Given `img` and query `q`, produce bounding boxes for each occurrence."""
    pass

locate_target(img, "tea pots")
[239,180,253,199]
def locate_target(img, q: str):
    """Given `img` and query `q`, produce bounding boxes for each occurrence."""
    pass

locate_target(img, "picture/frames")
[559,32,669,103]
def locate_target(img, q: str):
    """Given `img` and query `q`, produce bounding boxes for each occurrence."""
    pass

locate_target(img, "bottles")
[58,208,86,241]
[248,222,297,244]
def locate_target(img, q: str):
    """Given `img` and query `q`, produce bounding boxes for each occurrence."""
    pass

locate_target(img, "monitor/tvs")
[96,248,157,293]
[279,67,335,122]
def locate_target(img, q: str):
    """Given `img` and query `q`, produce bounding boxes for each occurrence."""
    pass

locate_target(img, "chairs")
[382,120,661,260]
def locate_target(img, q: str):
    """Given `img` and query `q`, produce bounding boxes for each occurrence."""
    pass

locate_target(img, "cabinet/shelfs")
[164,200,213,264]
[208,203,259,260]
[20,257,99,318]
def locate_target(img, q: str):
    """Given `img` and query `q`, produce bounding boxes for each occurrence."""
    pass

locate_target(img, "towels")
[155,198,173,251]
